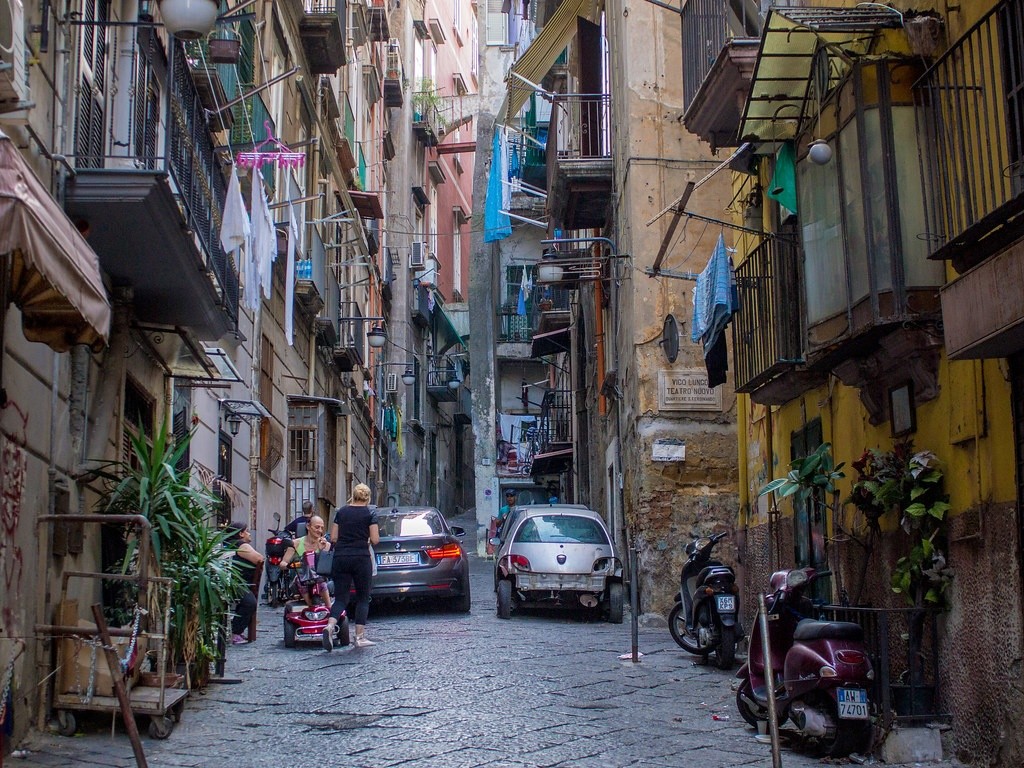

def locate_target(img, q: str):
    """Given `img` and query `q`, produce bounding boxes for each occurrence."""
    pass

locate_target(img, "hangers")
[237,121,306,170]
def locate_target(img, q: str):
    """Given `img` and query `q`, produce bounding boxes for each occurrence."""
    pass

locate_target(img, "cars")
[491,503,590,592]
[488,508,625,625]
[350,506,471,620]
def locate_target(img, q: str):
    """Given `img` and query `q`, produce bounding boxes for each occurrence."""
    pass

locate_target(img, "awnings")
[504,0,606,148]
[434,295,467,352]
[0,128,111,355]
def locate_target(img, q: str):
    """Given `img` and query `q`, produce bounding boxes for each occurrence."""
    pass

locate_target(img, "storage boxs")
[52,600,148,699]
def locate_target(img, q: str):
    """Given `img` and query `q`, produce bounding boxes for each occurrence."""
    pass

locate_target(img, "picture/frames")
[887,378,918,439]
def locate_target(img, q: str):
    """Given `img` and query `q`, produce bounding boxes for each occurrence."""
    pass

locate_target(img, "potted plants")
[80,402,258,694]
[385,67,401,79]
[538,297,553,311]
[755,438,956,726]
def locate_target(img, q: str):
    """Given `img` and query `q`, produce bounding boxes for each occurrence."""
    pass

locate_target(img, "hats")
[505,489,516,495]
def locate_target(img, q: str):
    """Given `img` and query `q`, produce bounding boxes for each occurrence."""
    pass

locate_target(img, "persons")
[495,489,516,537]
[279,516,332,607]
[322,483,380,653]
[217,521,264,645]
[282,501,315,539]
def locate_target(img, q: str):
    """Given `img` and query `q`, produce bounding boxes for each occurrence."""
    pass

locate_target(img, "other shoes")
[354,639,377,647]
[240,632,249,640]
[323,625,333,652]
[232,634,248,645]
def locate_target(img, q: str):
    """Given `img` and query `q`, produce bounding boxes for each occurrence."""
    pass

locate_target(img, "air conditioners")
[389,38,401,55]
[386,373,397,391]
[0,0,26,101]
[414,259,438,287]
[411,241,425,266]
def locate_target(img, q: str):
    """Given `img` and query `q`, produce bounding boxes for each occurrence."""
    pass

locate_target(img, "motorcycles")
[668,531,745,671]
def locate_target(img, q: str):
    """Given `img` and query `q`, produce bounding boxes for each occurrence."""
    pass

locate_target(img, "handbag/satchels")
[369,543,378,577]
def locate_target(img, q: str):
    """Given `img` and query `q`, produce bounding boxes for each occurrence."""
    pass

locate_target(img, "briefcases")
[314,549,335,578]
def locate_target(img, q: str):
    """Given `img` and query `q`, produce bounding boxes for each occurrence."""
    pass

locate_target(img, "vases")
[299,11,347,76]
[207,40,240,65]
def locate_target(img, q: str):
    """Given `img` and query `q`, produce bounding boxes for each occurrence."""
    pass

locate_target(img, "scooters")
[281,537,351,648]
[264,510,294,607]
[735,567,869,759]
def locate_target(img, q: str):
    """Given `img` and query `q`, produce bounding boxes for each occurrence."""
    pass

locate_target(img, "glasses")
[506,494,515,498]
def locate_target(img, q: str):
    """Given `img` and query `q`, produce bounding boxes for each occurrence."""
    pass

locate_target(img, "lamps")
[338,317,387,348]
[428,369,461,389]
[225,410,243,437]
[377,363,416,386]
[808,64,833,166]
[156,0,220,42]
[537,238,617,282]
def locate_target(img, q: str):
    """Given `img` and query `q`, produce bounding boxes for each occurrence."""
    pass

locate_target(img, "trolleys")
[34,512,190,742]
